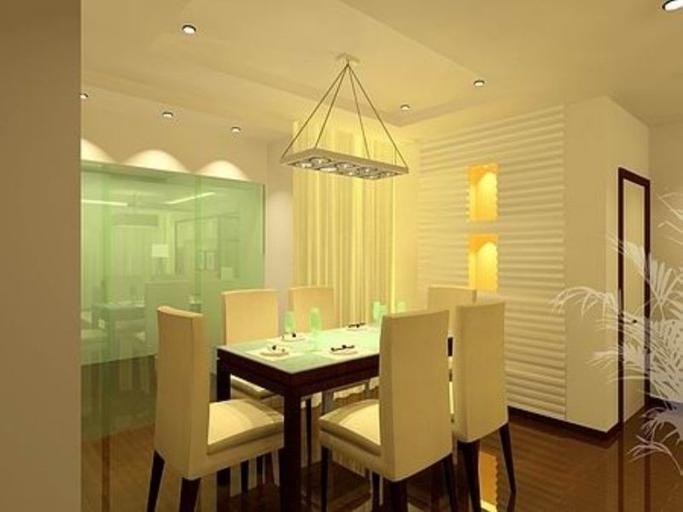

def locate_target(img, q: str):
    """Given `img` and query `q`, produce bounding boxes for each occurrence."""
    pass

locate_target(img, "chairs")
[77,271,245,417]
[426,284,478,311]
[289,284,371,414]
[219,286,315,512]
[320,306,461,512]
[451,298,516,512]
[146,305,285,512]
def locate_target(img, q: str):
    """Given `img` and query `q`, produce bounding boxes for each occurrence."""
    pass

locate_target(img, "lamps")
[279,61,409,181]
